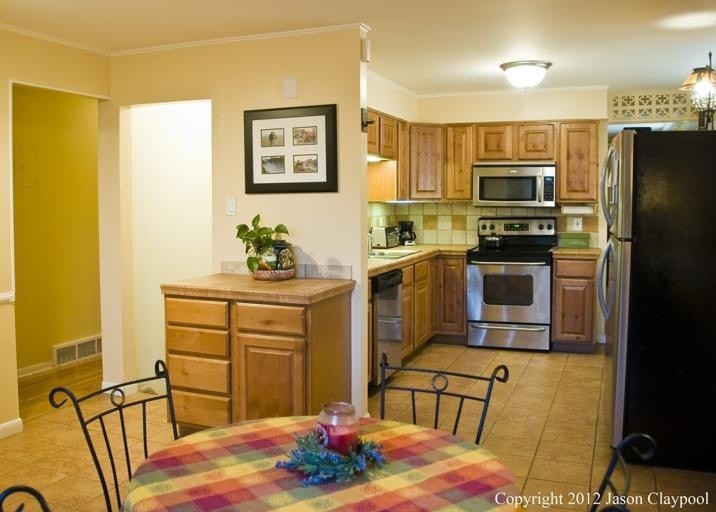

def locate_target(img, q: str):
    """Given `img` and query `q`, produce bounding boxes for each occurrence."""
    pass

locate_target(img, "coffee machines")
[398,220,415,245]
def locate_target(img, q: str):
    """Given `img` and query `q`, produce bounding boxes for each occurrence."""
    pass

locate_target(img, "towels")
[562,206,594,215]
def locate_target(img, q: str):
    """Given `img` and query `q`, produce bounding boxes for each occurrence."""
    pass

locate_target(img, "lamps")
[500,58,552,91]
[677,64,716,98]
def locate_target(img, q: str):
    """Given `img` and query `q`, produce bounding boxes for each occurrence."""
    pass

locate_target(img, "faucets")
[368,232,374,252]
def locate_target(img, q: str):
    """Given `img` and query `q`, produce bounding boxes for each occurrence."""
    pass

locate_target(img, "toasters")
[373,225,400,248]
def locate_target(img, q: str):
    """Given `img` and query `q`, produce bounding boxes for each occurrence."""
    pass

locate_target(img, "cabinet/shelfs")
[365,106,397,162]
[408,121,445,203]
[555,119,600,203]
[368,115,408,203]
[445,122,474,203]
[552,258,600,355]
[474,119,556,162]
[157,272,355,437]
[433,252,468,336]
[414,260,433,350]
[399,264,414,359]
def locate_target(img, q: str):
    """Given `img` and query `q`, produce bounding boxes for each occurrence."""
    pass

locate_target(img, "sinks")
[368,249,424,260]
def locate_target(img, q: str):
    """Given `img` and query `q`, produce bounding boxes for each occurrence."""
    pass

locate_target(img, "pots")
[484,232,522,247]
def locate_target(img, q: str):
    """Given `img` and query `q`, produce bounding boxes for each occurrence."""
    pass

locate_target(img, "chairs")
[48,355,176,512]
[0,485,50,512]
[579,428,659,509]
[378,354,508,446]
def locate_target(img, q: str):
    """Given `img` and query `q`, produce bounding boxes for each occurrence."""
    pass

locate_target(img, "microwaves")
[472,160,556,207]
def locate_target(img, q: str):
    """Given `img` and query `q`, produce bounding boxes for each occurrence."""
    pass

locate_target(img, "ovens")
[467,257,552,354]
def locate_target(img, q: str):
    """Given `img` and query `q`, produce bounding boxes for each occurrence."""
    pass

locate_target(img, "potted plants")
[234,212,298,280]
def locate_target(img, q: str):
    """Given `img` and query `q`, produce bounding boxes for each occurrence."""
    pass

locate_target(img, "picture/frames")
[241,104,340,193]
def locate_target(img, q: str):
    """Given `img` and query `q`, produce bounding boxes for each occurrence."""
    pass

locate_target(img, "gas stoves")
[471,244,556,256]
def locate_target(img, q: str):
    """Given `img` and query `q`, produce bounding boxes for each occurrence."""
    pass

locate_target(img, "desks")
[123,412,526,512]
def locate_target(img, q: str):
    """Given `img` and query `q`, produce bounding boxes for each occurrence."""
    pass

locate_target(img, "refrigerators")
[593,126,716,474]
